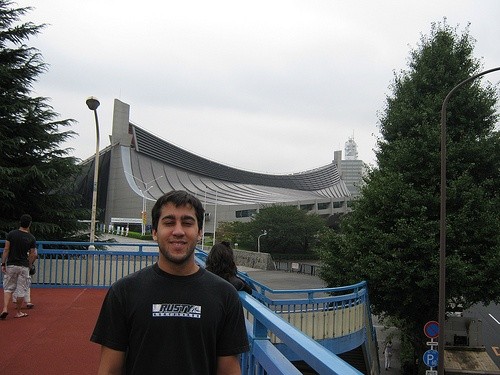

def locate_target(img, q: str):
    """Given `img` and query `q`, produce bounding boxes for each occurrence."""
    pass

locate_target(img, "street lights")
[258,233,267,252]
[85,94,101,286]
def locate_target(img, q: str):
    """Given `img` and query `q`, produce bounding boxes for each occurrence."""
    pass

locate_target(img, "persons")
[89,190,250,375]
[383,337,393,370]
[204,241,253,296]
[0,214,37,320]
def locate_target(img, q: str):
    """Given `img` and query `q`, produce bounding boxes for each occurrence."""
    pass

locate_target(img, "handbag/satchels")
[29,264,36,275]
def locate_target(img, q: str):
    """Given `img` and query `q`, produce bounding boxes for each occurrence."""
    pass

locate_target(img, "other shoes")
[26,303,34,307]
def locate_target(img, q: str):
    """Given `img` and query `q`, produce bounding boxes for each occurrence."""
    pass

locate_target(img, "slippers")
[14,313,28,318]
[0,312,8,320]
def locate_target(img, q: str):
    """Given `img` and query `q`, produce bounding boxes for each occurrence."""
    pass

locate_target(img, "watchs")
[1,262,5,266]
